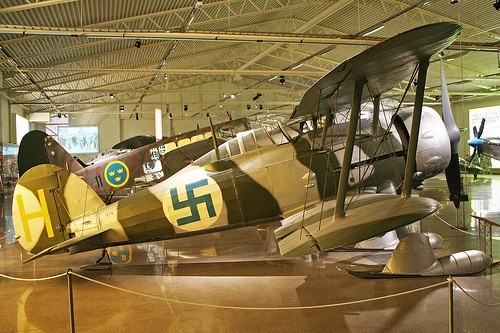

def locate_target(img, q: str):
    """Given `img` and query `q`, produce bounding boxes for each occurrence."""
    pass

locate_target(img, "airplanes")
[466,117,500,170]
[11,20,493,279]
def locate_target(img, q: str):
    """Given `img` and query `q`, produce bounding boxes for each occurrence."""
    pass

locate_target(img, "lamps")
[280,76,285,85]
[135,40,141,47]
[247,93,263,110]
